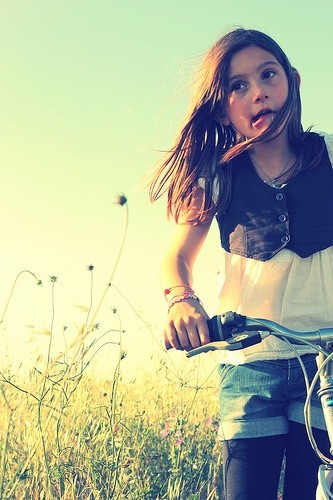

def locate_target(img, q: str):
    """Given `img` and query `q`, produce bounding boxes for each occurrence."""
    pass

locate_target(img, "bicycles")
[166,310,332,499]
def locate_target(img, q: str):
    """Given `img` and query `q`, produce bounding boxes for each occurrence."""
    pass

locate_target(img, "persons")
[148,24,333,500]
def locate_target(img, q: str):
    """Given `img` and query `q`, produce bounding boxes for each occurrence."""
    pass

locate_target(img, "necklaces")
[250,144,294,188]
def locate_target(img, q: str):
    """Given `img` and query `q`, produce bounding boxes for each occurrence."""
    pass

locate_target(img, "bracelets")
[164,292,201,311]
[164,285,195,299]
